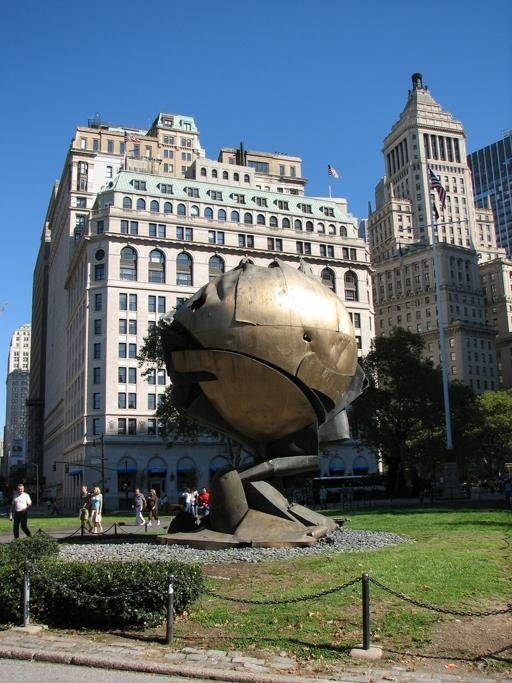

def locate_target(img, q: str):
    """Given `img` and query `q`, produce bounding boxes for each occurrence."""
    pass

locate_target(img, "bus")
[313,473,388,501]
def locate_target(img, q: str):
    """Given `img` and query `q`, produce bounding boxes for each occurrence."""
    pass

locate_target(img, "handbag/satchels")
[85,501,92,509]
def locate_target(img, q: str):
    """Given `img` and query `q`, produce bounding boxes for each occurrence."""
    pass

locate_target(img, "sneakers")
[136,521,160,526]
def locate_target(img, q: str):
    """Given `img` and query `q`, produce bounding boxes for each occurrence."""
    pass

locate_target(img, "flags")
[328,164,339,179]
[429,169,447,209]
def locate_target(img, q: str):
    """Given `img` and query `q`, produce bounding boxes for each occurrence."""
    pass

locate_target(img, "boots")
[94,526,102,535]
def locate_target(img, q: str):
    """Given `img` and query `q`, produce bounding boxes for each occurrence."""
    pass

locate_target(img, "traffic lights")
[52,464,56,471]
[65,463,69,472]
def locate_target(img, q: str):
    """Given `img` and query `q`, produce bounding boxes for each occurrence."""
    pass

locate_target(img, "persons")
[181,487,210,517]
[9,484,32,540]
[79,485,103,534]
[132,488,161,526]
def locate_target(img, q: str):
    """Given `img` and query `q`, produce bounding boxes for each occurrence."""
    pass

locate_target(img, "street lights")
[16,457,41,509]
[90,431,105,512]
